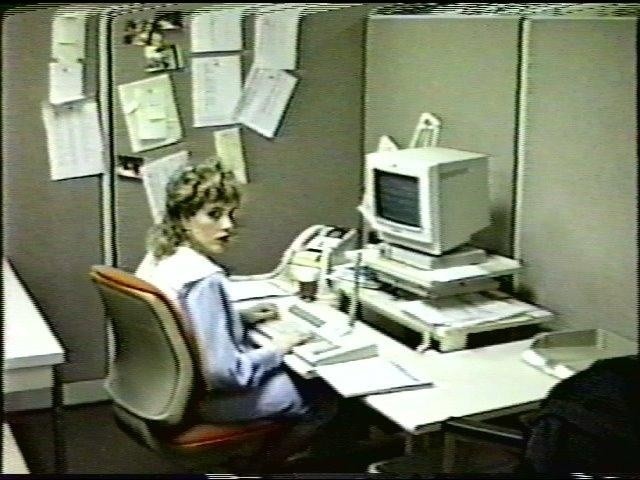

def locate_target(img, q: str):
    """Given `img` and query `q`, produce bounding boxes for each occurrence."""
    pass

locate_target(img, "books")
[312,353,437,398]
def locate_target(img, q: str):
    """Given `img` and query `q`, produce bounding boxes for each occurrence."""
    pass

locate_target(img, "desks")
[1,253,70,475]
[224,249,580,451]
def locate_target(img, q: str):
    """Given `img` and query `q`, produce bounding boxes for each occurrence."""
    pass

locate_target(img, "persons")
[135,158,338,471]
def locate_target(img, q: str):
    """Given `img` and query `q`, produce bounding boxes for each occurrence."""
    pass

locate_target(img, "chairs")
[367,417,527,473]
[88,265,303,474]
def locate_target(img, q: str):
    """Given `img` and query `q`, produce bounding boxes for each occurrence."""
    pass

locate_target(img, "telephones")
[283,222,355,272]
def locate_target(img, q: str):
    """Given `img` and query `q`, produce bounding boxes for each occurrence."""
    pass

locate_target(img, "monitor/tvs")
[364,147,492,271]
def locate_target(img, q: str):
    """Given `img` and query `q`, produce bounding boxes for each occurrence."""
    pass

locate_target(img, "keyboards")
[252,296,378,367]
